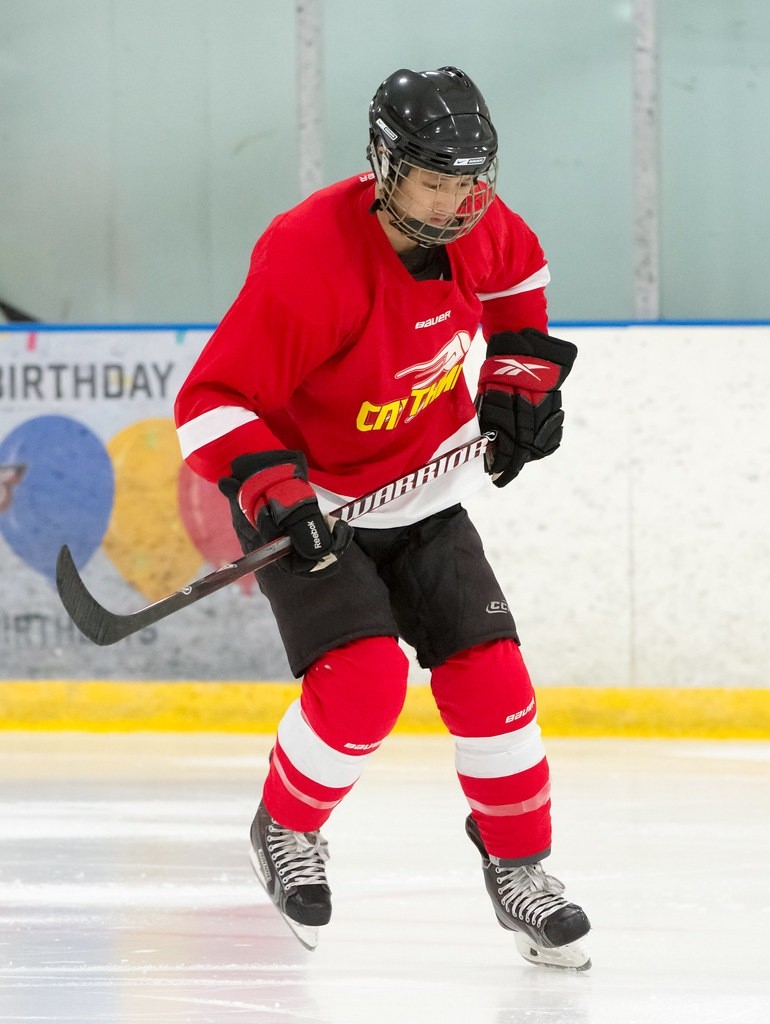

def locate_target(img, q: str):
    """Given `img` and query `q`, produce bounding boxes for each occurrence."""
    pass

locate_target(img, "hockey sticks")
[50,424,508,651]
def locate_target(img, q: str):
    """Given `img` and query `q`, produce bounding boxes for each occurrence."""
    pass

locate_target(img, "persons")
[173,65,592,947]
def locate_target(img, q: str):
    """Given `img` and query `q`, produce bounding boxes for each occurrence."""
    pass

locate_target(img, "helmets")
[365,65,498,250]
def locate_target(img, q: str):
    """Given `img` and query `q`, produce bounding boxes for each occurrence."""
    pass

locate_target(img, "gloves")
[218,450,353,581]
[472,328,578,489]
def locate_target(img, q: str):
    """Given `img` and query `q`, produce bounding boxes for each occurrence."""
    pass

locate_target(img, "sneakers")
[463,812,593,972]
[247,798,335,952]
